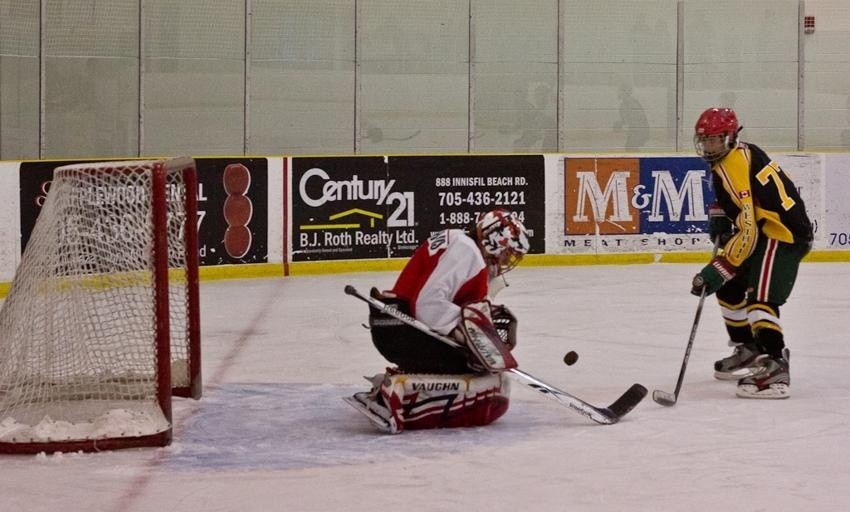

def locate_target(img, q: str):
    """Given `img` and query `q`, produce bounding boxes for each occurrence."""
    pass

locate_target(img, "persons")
[353,209,532,435]
[688,105,817,393]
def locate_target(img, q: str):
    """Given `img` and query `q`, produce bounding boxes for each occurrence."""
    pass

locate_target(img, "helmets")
[695,107,740,148]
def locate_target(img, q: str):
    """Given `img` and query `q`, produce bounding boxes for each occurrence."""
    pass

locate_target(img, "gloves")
[452,298,518,373]
[690,256,736,297]
[709,205,734,246]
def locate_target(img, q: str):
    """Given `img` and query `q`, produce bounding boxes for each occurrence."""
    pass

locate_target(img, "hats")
[473,209,531,257]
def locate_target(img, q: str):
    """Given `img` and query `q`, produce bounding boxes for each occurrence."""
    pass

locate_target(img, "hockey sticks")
[344,285,648,424]
[652,235,720,406]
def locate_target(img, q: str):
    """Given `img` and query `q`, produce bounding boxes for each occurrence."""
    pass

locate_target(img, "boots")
[713,340,791,389]
[355,367,413,435]
